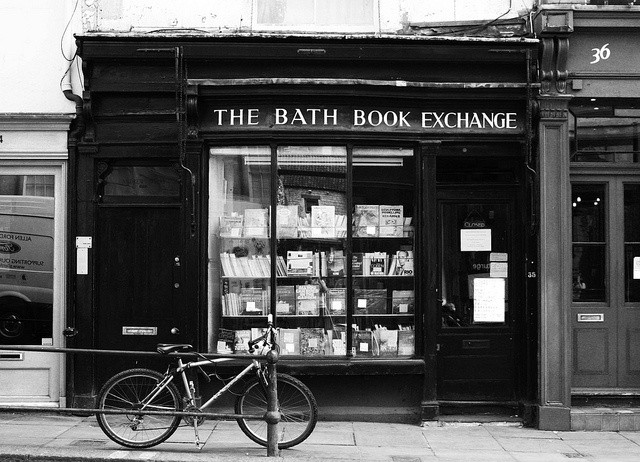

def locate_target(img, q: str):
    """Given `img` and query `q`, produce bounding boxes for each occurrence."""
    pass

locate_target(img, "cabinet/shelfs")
[201,134,420,376]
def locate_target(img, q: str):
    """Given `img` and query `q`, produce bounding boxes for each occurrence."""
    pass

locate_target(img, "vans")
[1,195,55,343]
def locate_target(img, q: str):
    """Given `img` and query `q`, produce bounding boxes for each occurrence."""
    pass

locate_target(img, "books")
[356,326,359,330]
[398,324,415,330]
[404,217,413,237]
[311,205,335,238]
[379,205,403,238]
[217,351,233,354]
[379,325,381,328]
[262,286,271,316]
[301,328,325,357]
[313,251,328,277]
[375,324,378,330]
[352,324,356,330]
[388,254,397,275]
[354,204,379,237]
[386,255,389,274]
[298,213,311,238]
[327,330,332,355]
[244,208,269,237]
[296,285,319,315]
[234,330,250,351]
[336,214,347,238]
[221,292,240,315]
[365,328,371,331]
[219,252,287,277]
[276,205,298,238]
[341,332,345,356]
[219,214,244,238]
[364,251,386,275]
[276,285,296,314]
[396,251,414,276]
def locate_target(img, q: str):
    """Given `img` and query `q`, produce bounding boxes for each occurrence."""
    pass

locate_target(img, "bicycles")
[95,313,318,449]
